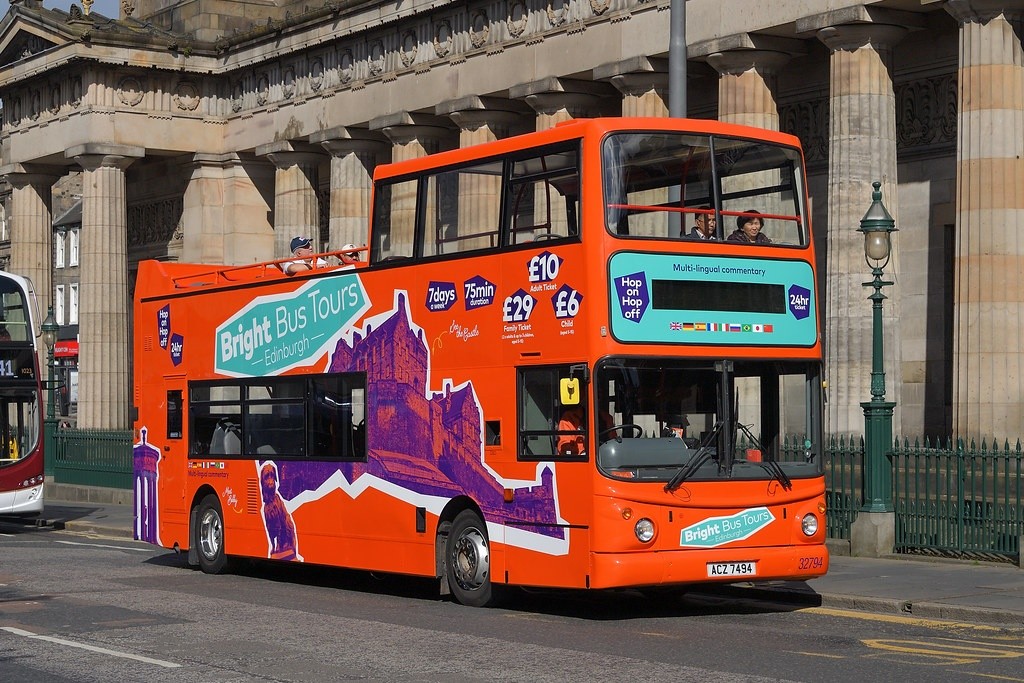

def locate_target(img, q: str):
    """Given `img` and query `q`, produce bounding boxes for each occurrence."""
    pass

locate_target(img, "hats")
[342,244,360,255]
[291,236,313,253]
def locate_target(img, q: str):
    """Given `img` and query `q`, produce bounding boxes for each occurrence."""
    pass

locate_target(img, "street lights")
[856,181,900,512]
[40,305,60,477]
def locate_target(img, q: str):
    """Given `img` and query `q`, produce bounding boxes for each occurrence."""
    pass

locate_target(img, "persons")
[60,422,77,460]
[340,244,361,265]
[727,210,771,243]
[283,237,329,274]
[2,425,19,461]
[557,385,617,455]
[681,206,719,240]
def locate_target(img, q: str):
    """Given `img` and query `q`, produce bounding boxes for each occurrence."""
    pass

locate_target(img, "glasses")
[697,218,716,224]
[299,243,313,249]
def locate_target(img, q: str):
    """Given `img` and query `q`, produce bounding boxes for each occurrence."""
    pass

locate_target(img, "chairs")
[207,417,365,458]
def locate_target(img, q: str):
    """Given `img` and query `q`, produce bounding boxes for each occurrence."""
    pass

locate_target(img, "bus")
[133,118,828,606]
[0,270,65,523]
